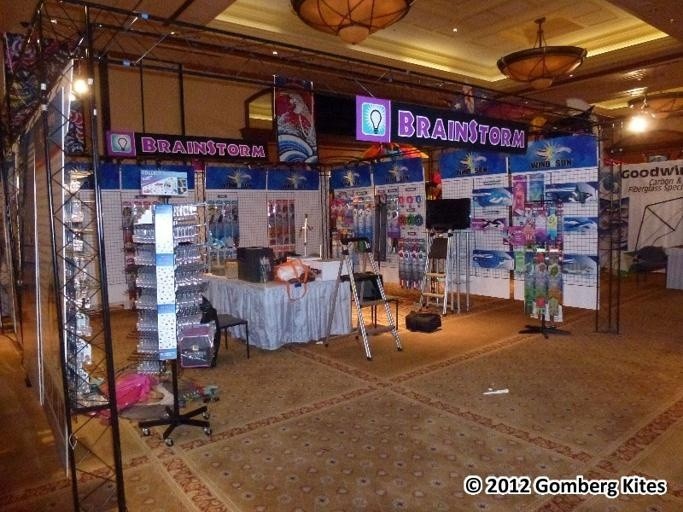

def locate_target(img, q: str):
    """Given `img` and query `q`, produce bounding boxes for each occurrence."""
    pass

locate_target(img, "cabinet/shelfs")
[131,203,212,445]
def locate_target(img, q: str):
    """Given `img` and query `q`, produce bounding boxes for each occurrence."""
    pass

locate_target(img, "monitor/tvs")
[426,197,471,230]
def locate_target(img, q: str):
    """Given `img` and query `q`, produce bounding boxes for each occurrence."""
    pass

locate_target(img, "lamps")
[291,0,414,47]
[497,17,587,91]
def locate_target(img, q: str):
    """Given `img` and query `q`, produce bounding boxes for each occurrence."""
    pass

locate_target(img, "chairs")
[199,295,250,366]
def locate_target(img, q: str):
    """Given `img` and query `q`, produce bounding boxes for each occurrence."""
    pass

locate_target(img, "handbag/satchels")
[405,311,441,333]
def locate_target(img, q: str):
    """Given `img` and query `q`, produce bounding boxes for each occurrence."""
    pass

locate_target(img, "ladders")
[324,237,403,361]
[418,232,454,317]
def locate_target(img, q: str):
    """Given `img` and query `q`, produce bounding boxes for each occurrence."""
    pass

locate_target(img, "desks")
[207,273,350,350]
